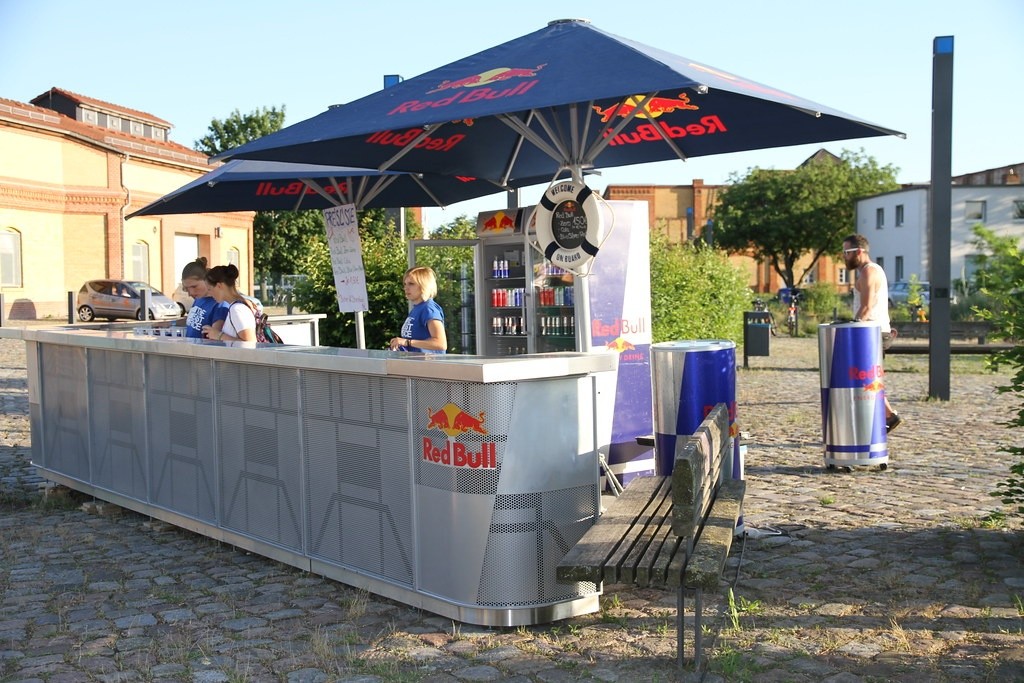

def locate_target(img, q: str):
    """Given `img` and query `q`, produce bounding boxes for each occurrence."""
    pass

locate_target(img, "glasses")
[843,248,864,255]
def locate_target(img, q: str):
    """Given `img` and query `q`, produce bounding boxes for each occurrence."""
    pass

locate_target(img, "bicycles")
[749,296,778,336]
[783,293,799,337]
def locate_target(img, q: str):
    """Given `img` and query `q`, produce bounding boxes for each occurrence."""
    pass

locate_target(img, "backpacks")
[229,298,284,344]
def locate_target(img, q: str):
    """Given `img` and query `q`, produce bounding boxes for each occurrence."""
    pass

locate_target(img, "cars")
[886,282,931,308]
[76,279,182,321]
[172,282,264,318]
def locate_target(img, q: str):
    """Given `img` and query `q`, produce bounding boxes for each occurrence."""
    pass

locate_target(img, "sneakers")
[886,410,904,434]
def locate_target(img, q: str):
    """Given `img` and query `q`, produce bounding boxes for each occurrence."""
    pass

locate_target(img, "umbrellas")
[206,20,909,353]
[125,104,602,350]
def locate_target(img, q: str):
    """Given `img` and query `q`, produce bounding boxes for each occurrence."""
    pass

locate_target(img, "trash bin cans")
[778,288,792,303]
[650,339,742,480]
[819,321,890,473]
[744,311,770,356]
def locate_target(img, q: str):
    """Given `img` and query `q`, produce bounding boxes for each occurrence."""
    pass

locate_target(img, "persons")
[201,264,260,342]
[389,266,448,355]
[841,234,902,434]
[121,287,131,297]
[181,258,230,340]
[112,285,119,295]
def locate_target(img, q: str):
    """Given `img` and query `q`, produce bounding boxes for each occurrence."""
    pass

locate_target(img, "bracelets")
[217,331,224,341]
[407,339,411,347]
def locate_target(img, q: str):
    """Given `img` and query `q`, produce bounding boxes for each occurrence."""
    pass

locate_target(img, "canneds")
[649,338,745,536]
[817,318,889,467]
[539,257,574,336]
[493,259,527,336]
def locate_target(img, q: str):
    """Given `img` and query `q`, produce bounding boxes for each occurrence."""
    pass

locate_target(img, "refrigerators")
[407,200,656,493]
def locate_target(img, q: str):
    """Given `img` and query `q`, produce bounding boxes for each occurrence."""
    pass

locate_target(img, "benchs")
[890,320,1017,375]
[555,402,745,683]
